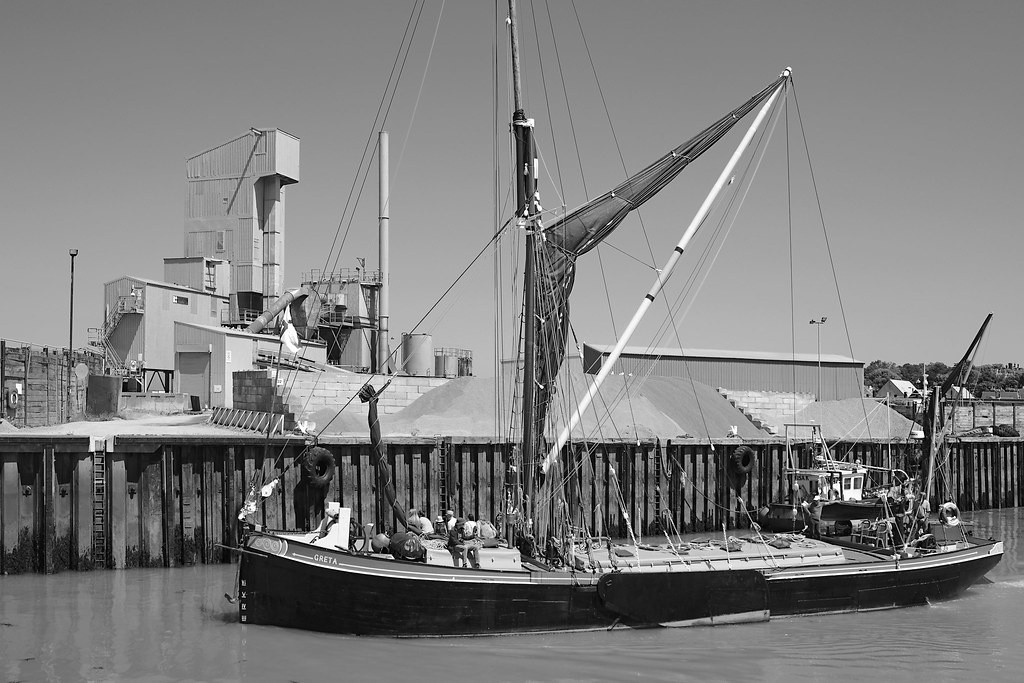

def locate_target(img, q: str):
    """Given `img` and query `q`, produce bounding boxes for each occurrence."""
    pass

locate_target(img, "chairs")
[850,520,890,548]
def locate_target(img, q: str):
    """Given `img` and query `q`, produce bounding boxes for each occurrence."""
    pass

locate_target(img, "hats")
[813,496,820,500]
[435,516,444,522]
[445,511,453,515]
[455,519,466,525]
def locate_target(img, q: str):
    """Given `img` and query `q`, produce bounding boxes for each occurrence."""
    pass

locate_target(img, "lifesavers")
[732,445,758,474]
[938,503,960,527]
[302,447,336,489]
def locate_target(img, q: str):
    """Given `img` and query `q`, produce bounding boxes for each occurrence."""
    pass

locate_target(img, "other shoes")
[475,564,479,568]
[463,563,467,567]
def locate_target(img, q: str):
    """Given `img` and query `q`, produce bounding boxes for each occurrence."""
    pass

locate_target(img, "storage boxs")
[938,542,956,553]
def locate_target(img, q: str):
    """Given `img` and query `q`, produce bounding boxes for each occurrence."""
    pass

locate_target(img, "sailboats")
[237,0,1004,637]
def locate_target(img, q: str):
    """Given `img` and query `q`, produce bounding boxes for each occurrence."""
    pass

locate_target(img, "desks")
[140,366,175,393]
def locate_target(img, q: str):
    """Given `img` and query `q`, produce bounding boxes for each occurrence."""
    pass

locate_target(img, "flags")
[279,304,303,354]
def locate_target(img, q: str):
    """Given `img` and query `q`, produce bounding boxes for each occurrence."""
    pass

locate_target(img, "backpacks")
[480,520,492,537]
[436,523,446,533]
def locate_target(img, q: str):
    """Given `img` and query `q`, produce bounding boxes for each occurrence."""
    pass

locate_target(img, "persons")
[407,508,500,569]
[915,492,931,548]
[805,495,841,539]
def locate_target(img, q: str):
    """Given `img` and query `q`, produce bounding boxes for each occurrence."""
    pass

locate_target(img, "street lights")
[808,316,827,402]
[64,248,82,419]
